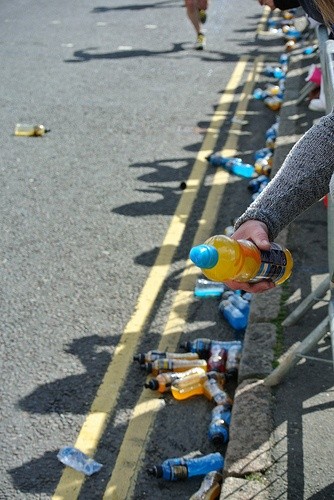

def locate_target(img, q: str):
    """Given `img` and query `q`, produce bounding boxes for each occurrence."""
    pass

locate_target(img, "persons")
[259,0,334,39]
[185,0,208,50]
[224,111,334,294]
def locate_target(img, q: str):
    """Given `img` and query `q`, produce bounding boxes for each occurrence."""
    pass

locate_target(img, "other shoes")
[198,9,206,24]
[194,34,205,49]
